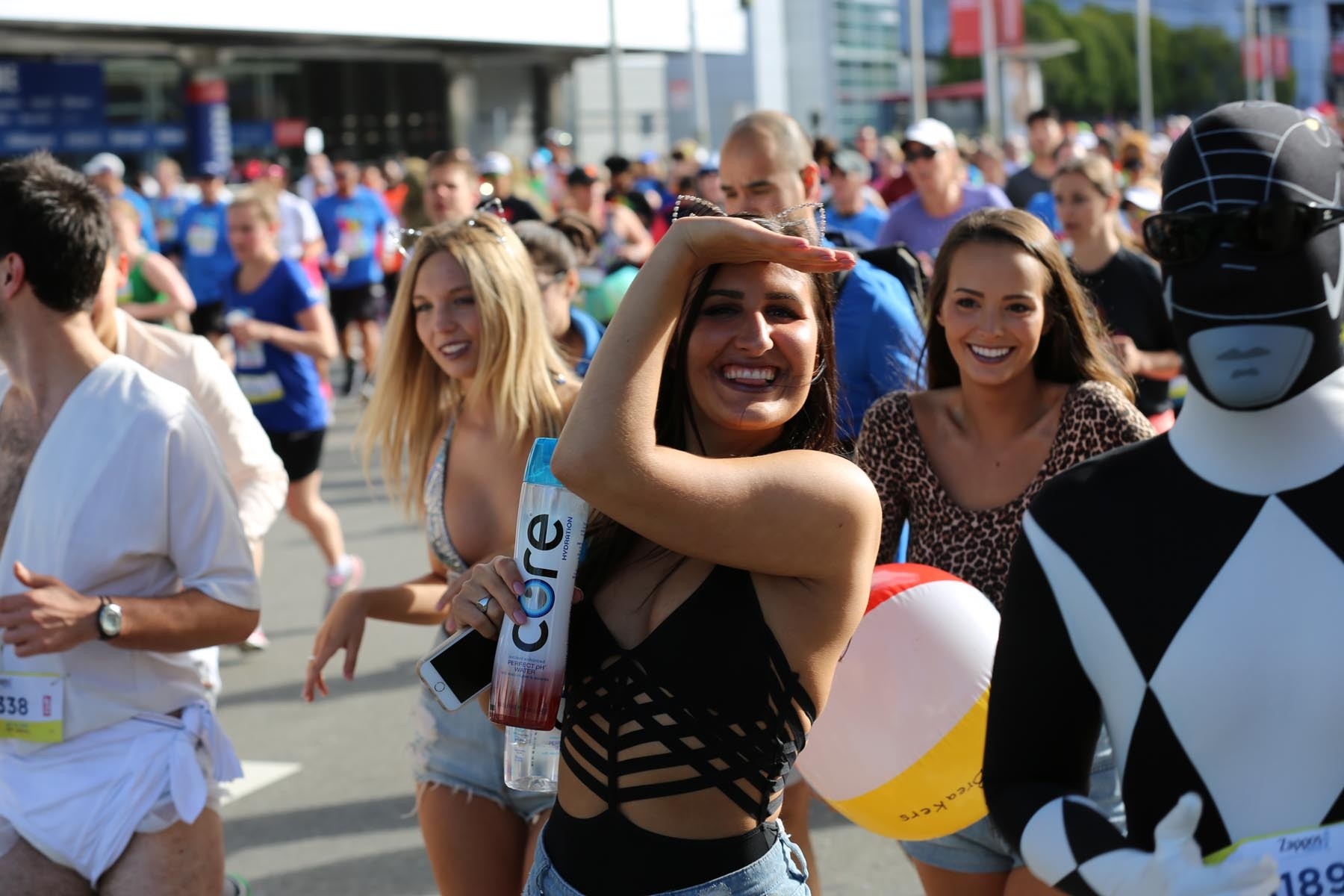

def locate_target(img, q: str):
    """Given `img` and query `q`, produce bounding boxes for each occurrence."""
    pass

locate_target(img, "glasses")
[1120,158,1148,174]
[905,147,951,161]
[1142,200,1344,270]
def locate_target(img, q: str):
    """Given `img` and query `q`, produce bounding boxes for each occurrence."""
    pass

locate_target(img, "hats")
[900,118,956,147]
[83,152,124,179]
[476,150,511,177]
[828,145,872,180]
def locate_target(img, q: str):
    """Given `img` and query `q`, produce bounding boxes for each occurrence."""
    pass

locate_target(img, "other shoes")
[240,630,268,651]
[326,554,366,617]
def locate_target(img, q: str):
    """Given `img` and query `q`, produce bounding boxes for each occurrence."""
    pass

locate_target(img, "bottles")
[487,437,589,731]
[503,687,566,793]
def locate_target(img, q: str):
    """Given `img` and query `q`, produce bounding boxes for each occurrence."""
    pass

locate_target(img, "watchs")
[95,593,123,644]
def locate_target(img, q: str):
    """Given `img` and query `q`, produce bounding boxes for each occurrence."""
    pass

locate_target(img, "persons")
[0,95,1344,896]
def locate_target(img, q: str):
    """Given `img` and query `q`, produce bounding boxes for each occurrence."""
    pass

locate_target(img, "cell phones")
[416,621,500,713]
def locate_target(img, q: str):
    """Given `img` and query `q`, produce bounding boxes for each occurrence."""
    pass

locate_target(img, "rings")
[477,594,492,612]
